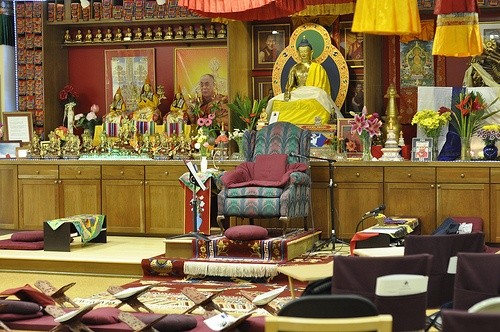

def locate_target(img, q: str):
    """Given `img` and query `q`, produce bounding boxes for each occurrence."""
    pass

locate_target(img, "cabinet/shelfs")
[46,17,228,46]
[235,167,500,244]
[0,164,235,236]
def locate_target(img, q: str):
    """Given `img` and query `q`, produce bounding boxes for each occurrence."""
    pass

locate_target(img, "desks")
[349,215,421,255]
[43,213,107,251]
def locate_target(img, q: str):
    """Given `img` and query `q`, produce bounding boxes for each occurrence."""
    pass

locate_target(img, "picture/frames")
[336,118,363,160]
[338,20,365,114]
[411,137,434,162]
[251,24,289,99]
[104,47,227,111]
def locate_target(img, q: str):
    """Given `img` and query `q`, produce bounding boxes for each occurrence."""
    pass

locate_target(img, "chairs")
[215,121,311,237]
[263,232,500,332]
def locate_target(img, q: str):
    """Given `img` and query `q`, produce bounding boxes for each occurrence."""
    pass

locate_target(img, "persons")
[260,34,280,62]
[113,117,136,149]
[133,75,158,122]
[347,32,363,60]
[272,36,332,126]
[351,83,364,111]
[327,106,337,124]
[28,133,200,160]
[164,87,188,124]
[257,108,267,122]
[106,87,127,123]
[267,89,272,96]
[184,74,229,130]
[64,24,226,43]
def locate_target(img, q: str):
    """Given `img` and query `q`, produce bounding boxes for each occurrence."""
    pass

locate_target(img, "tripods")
[169,162,211,243]
[306,155,353,252]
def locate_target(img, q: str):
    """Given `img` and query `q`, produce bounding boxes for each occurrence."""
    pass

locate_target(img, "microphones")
[364,205,386,215]
[289,151,307,159]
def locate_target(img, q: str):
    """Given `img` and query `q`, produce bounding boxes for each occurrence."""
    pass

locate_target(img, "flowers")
[192,91,271,149]
[410,88,500,146]
[58,83,102,132]
[348,105,382,137]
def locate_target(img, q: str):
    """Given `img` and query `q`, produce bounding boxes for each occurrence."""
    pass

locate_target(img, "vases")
[460,137,472,161]
[484,141,499,161]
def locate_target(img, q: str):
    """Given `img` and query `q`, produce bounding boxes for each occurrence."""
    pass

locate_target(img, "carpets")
[73,230,341,319]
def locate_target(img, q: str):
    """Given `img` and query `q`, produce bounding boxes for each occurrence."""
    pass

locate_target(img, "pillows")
[254,154,288,182]
